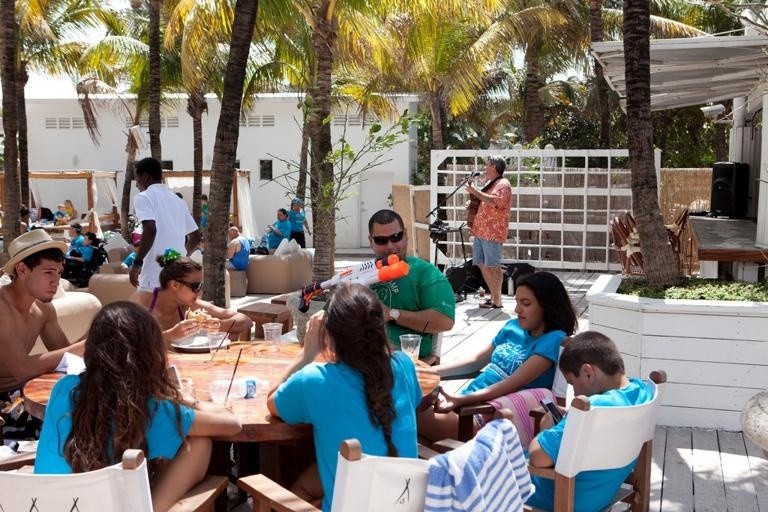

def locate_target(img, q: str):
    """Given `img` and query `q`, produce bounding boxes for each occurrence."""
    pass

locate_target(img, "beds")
[159,165,257,235]
[22,170,120,233]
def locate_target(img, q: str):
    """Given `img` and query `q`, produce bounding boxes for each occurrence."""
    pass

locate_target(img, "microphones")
[461,173,481,181]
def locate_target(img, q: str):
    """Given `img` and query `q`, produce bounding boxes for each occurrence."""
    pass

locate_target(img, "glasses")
[174,278,200,292]
[371,231,403,244]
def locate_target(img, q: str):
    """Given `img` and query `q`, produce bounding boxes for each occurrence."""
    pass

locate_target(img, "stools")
[273,295,293,307]
[237,299,293,347]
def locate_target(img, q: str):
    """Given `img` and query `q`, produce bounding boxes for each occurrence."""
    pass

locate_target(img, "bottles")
[210,378,269,402]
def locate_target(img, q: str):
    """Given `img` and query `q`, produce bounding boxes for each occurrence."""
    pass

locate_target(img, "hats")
[2,228,67,276]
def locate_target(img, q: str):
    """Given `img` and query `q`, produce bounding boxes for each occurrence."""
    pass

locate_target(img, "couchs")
[223,269,247,303]
[246,247,317,295]
[90,261,162,304]
[29,277,102,354]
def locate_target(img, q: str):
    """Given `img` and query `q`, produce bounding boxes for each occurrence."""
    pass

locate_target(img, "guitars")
[465,173,481,231]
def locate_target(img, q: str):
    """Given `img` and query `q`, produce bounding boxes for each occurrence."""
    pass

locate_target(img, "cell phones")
[540,398,563,424]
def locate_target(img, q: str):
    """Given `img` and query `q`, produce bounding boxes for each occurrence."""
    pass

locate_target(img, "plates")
[169,332,232,353]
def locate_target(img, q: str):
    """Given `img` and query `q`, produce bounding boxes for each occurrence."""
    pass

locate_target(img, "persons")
[264,208,291,255]
[318,210,457,359]
[0,229,90,441]
[225,226,251,271]
[465,155,511,308]
[64,198,78,221]
[121,240,140,272]
[287,198,311,248]
[66,223,85,257]
[128,157,202,288]
[63,232,98,276]
[200,194,208,255]
[52,205,71,226]
[20,203,30,235]
[524,331,655,512]
[247,284,423,512]
[128,249,253,345]
[33,300,242,512]
[417,271,578,447]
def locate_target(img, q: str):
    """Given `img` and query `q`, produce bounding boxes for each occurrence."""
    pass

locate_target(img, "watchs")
[133,259,144,267]
[389,308,400,320]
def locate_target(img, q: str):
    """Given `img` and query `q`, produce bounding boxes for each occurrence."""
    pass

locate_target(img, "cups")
[206,331,230,365]
[399,333,422,364]
[209,370,240,406]
[261,322,284,351]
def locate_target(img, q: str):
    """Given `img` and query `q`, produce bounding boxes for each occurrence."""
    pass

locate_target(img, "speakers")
[710,162,750,219]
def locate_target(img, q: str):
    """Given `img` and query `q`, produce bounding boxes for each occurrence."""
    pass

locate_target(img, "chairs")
[0,453,229,512]
[425,336,574,440]
[432,367,669,512]
[237,417,514,512]
